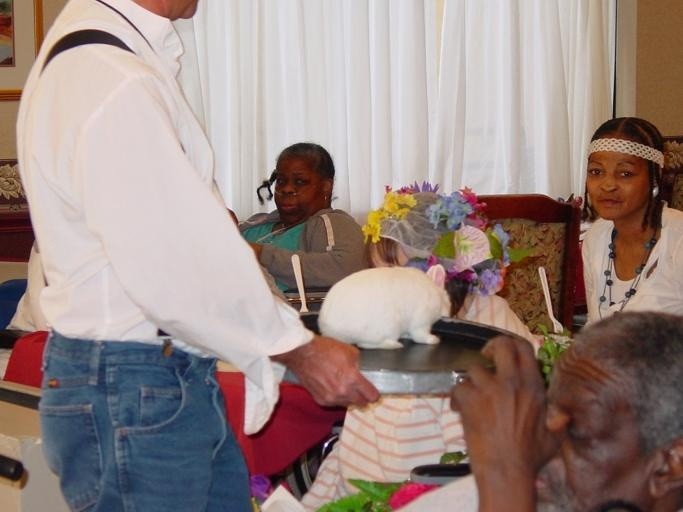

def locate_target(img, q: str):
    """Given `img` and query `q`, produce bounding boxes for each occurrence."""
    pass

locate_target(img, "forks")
[537,266,564,334]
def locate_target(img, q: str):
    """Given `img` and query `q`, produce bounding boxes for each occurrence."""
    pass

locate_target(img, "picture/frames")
[0,0,44,102]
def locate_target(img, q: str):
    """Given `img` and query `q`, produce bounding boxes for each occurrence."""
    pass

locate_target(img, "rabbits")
[317,262,453,352]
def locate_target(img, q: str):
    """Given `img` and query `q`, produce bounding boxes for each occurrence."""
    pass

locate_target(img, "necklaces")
[599,227,658,321]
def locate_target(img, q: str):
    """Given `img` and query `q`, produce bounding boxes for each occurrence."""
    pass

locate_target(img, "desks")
[3,331,347,482]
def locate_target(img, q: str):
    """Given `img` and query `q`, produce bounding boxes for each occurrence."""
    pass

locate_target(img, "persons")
[228,143,368,293]
[450,311,683,511]
[581,117,683,332]
[14,0,381,512]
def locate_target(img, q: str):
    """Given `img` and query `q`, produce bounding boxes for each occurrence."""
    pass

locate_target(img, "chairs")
[464,194,581,338]
[655,137,683,210]
